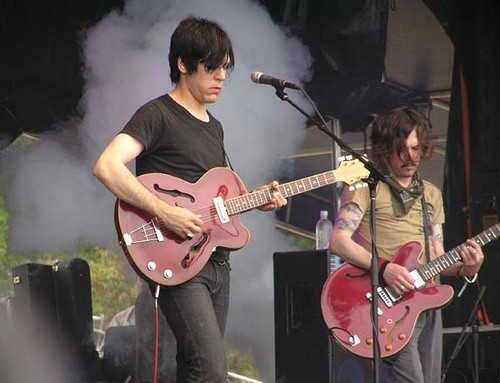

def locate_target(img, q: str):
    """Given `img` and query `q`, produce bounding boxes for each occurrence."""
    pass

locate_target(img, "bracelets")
[379,257,390,280]
[456,264,463,280]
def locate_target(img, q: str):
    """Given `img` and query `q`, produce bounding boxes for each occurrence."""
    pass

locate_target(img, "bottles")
[316,210,332,249]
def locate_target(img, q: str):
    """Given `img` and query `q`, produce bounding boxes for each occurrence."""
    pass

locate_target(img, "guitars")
[320,223,500,359]
[114,152,371,288]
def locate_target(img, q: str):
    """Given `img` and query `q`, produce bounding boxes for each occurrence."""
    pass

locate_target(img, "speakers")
[0,256,136,383]
[273,251,399,383]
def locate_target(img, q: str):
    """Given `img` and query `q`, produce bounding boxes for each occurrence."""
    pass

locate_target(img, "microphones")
[251,71,301,90]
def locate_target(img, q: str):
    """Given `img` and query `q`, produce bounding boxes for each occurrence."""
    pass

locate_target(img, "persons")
[93,17,289,383]
[330,104,483,383]
[97,278,145,357]
